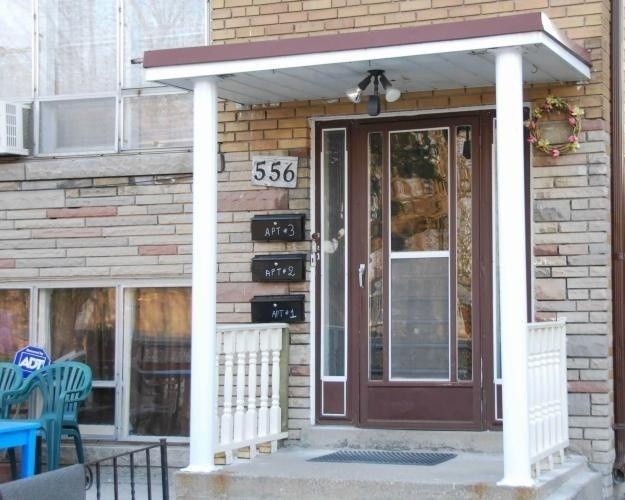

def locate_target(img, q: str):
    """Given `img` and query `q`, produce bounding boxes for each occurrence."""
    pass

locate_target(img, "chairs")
[0,361,92,482]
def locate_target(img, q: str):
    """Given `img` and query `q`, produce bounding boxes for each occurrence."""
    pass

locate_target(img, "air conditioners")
[1,98,31,155]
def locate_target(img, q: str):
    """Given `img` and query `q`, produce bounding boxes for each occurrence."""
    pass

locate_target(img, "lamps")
[345,63,401,115]
